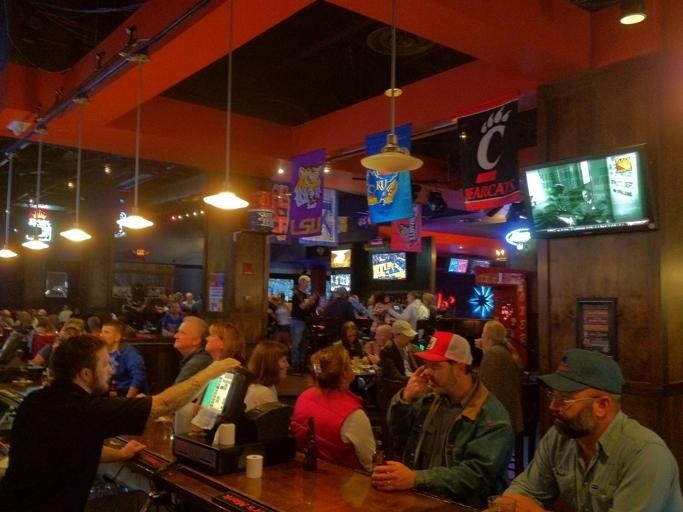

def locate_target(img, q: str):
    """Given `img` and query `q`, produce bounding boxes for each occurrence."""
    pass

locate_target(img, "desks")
[2,312,537,489]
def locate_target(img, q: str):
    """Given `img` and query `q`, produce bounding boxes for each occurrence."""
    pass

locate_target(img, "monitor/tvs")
[516,142,660,239]
[329,249,352,271]
[330,272,353,292]
[446,257,470,276]
[199,368,250,419]
[0,330,25,369]
[369,252,408,281]
[469,259,492,277]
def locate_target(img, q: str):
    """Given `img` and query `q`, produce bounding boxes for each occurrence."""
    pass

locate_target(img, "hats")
[411,331,475,367]
[533,347,626,397]
[389,319,418,337]
[62,318,84,331]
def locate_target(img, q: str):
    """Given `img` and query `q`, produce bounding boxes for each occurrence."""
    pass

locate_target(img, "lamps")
[49,95,96,245]
[111,46,159,232]
[355,0,426,178]
[618,0,647,26]
[0,142,19,261]
[197,0,254,211]
[17,122,52,250]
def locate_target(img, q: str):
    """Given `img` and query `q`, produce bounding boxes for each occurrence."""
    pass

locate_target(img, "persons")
[1,308,85,368]
[122,292,202,334]
[292,345,378,472]
[474,320,523,433]
[501,349,683,512]
[571,189,610,225]
[100,322,149,397]
[0,335,242,511]
[532,184,570,230]
[370,332,514,510]
[172,316,290,413]
[267,275,437,411]
